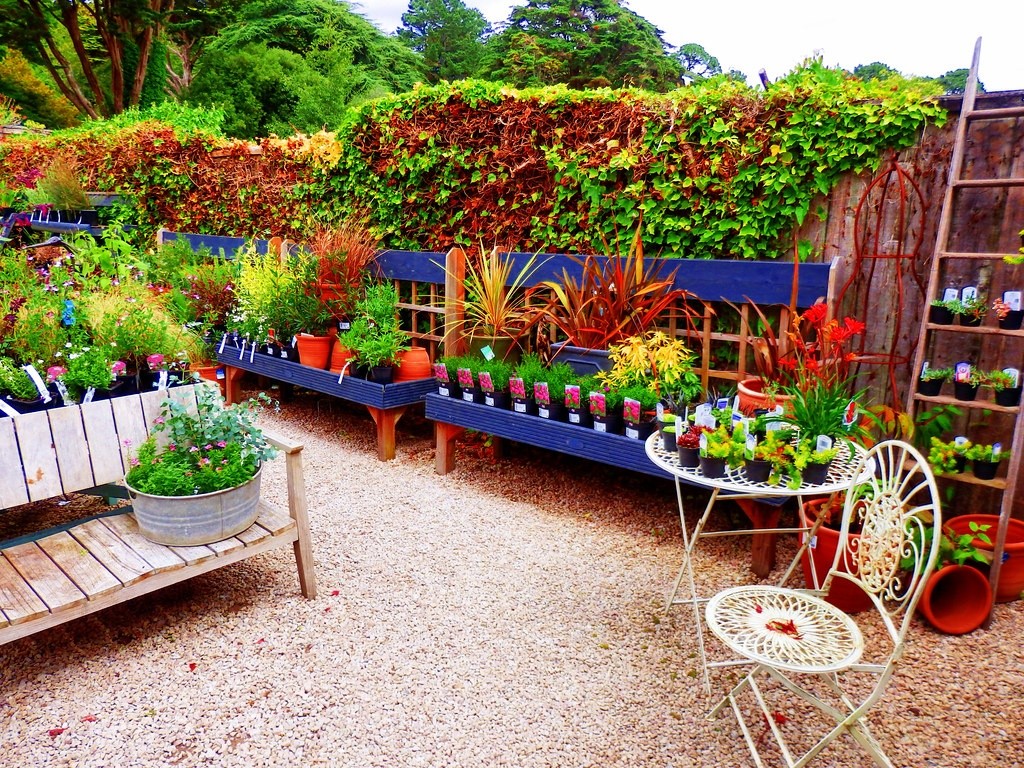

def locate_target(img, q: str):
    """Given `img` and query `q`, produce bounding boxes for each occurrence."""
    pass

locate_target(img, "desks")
[643,423,877,688]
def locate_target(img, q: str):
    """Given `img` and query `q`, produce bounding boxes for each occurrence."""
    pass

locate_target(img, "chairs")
[702,440,942,767]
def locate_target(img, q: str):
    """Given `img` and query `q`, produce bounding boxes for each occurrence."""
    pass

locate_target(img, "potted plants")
[917,367,958,396]
[992,297,1024,331]
[956,288,990,329]
[657,370,888,490]
[501,211,717,383]
[413,231,556,359]
[927,436,973,477]
[0,210,431,419]
[964,442,1013,481]
[118,353,276,547]
[718,292,868,416]
[795,473,994,615]
[927,289,960,325]
[981,368,1023,406]
[432,328,703,445]
[952,365,987,402]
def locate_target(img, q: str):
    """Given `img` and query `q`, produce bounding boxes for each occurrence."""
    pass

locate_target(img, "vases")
[943,512,1023,603]
[907,561,994,637]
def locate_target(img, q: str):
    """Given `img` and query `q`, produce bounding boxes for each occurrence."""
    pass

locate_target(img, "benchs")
[0,380,318,647]
